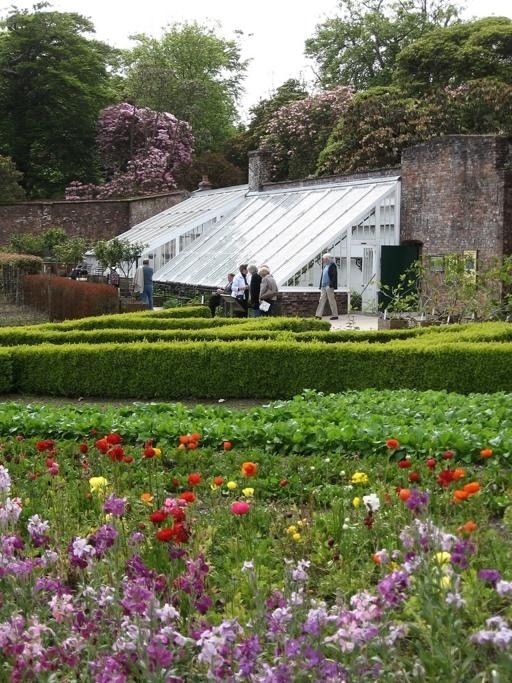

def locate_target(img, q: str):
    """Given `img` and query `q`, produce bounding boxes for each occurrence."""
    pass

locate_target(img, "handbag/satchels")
[261,284,279,300]
[236,295,243,302]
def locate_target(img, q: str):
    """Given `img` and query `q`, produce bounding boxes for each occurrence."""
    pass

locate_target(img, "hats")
[239,264,248,270]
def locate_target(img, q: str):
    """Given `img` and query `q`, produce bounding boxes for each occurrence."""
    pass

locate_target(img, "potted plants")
[369,251,512,331]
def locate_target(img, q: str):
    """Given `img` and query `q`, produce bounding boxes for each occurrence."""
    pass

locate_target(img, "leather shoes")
[315,316,321,320]
[330,317,338,320]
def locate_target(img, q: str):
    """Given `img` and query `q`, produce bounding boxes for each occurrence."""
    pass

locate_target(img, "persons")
[209,264,278,317]
[108,267,119,289]
[133,260,153,309]
[76,266,88,279]
[313,252,339,320]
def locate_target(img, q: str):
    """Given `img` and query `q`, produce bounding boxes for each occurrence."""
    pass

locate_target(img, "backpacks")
[107,266,118,285]
